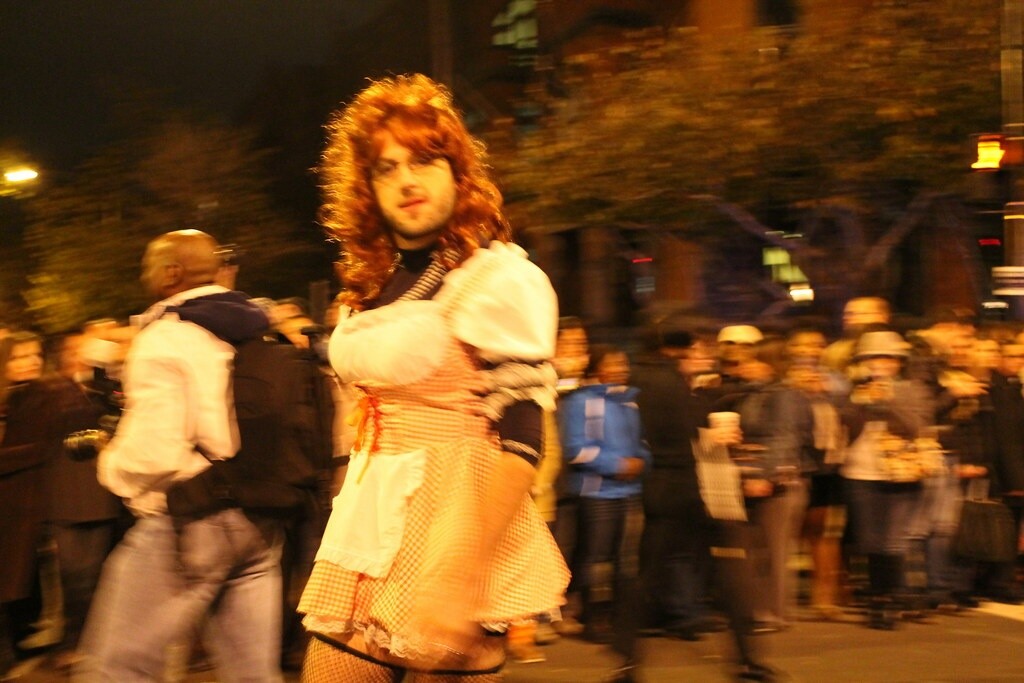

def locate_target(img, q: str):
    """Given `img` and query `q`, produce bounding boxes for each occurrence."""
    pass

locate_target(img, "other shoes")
[504,573,987,683]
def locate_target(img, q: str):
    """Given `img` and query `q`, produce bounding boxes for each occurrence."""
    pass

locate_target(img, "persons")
[509,296,1024,683]
[296,73,573,683]
[0,283,354,683]
[72,228,284,683]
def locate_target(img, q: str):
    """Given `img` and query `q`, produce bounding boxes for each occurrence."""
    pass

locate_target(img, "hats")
[718,324,765,346]
[851,332,913,362]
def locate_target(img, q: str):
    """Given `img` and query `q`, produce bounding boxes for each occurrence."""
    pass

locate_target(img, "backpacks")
[157,289,337,530]
